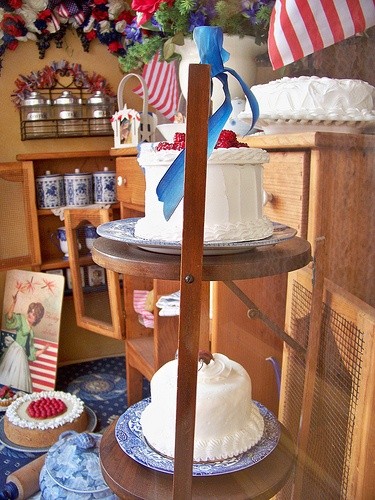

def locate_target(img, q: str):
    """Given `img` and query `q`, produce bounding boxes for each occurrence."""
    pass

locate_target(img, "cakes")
[4,390,87,448]
[139,351,265,463]
[135,129,274,243]
[241,75,375,122]
[0,385,16,406]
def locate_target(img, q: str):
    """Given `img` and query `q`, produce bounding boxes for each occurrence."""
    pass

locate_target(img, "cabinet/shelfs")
[60,127,375,413]
[0,151,118,308]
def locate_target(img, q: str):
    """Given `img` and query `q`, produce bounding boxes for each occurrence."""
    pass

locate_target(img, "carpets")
[0,356,151,500]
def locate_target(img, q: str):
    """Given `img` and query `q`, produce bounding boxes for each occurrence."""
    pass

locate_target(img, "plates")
[96,218,297,254]
[115,396,281,477]
[0,405,97,452]
[239,113,375,133]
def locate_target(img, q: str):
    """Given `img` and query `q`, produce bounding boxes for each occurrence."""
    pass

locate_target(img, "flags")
[131,50,183,124]
[268,0,375,72]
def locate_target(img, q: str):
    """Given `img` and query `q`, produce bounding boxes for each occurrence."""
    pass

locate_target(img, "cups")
[55,227,82,259]
[93,166,117,205]
[63,168,93,207]
[35,170,65,209]
[84,224,99,256]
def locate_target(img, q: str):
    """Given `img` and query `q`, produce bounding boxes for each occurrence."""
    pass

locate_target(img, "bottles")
[53,91,83,136]
[86,90,115,134]
[19,91,52,136]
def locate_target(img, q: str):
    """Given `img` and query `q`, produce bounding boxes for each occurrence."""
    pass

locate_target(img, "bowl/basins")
[156,118,256,141]
[38,431,121,500]
